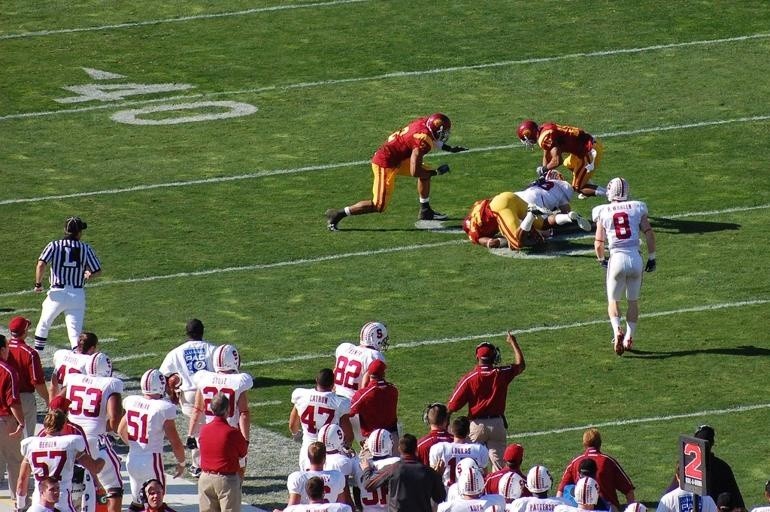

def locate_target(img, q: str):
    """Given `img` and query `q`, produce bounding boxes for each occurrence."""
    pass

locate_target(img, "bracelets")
[35,282,40,288]
[597,256,606,261]
[646,251,656,260]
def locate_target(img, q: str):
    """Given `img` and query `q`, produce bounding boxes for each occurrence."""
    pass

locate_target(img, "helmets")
[140,368,166,397]
[607,177,629,201]
[318,424,393,457]
[574,477,600,505]
[426,112,451,142]
[359,322,389,349]
[545,169,564,181]
[517,120,538,151]
[457,457,552,499]
[624,503,648,512]
[85,352,112,376]
[463,217,471,233]
[213,344,239,372]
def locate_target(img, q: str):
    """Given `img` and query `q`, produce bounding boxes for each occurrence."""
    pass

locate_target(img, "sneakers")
[527,202,553,216]
[187,465,201,476]
[418,208,448,220]
[326,209,338,231]
[569,211,593,232]
[611,327,633,355]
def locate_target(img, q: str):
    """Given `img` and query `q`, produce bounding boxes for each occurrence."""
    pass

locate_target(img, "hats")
[717,492,738,511]
[694,425,714,437]
[579,460,597,473]
[502,444,525,463]
[64,215,86,233]
[9,316,32,332]
[187,318,204,330]
[477,342,497,360]
[368,359,387,375]
[51,396,71,412]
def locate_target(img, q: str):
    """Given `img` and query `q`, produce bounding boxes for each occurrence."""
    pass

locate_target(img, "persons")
[461,192,591,252]
[415,403,636,511]
[1,316,254,512]
[323,113,469,233]
[506,168,576,216]
[34,216,103,351]
[285,358,447,511]
[592,176,657,354]
[332,322,389,447]
[654,425,770,512]
[447,331,526,471]
[515,120,608,199]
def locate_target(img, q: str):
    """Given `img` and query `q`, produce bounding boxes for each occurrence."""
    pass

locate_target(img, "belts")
[472,414,508,429]
[53,284,83,288]
[203,469,237,476]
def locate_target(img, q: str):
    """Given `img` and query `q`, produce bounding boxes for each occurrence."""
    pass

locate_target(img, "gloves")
[599,257,610,267]
[173,462,185,478]
[438,165,449,174]
[536,166,548,174]
[452,146,468,153]
[187,436,197,449]
[644,258,656,272]
